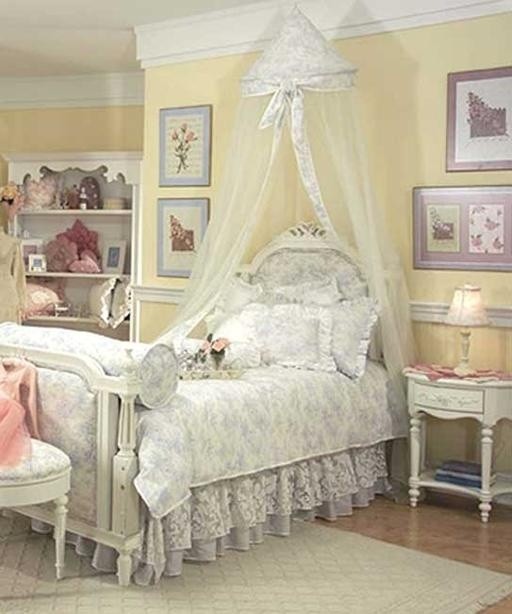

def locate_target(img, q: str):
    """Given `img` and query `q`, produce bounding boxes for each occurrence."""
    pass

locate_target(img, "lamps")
[444,283,491,377]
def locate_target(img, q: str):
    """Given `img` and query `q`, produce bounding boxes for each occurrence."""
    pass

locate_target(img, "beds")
[1,219,408,586]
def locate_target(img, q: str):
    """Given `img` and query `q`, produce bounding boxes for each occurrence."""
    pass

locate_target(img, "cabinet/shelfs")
[1,150,143,342]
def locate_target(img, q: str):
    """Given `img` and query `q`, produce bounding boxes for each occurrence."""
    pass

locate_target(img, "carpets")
[0,520,512,614]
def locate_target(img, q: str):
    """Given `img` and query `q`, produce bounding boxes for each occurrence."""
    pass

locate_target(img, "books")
[443,459,484,475]
[433,475,497,488]
[436,468,495,482]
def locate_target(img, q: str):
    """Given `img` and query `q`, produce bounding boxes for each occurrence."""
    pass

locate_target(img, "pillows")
[110,276,131,329]
[274,277,344,306]
[325,296,379,380]
[260,302,337,372]
[87,278,117,330]
[210,303,266,370]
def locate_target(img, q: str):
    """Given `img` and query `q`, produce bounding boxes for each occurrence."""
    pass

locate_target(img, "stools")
[0,438,71,579]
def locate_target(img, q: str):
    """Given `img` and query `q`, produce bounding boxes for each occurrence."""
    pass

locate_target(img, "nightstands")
[403,366,512,523]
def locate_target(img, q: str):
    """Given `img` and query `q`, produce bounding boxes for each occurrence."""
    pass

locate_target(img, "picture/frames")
[102,240,126,274]
[158,104,212,188]
[412,184,512,273]
[21,238,44,269]
[156,197,210,278]
[446,66,512,172]
[28,254,47,273]
